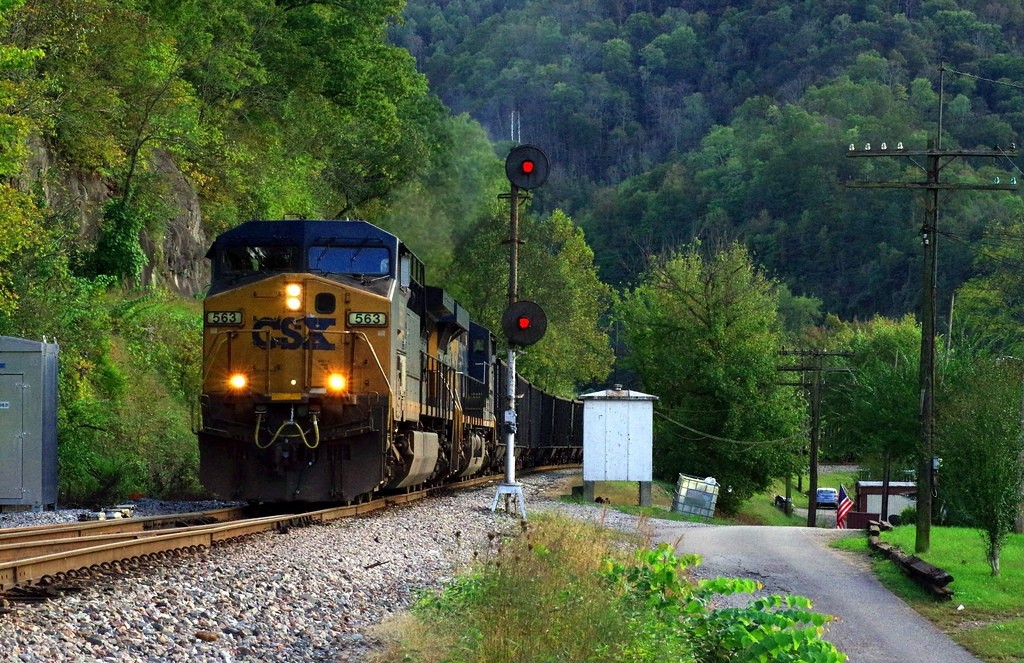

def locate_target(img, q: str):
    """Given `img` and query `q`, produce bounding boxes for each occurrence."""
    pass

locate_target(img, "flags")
[836,484,856,528]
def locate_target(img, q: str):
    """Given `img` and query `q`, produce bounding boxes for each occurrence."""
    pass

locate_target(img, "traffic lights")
[502,301,548,347]
[505,145,549,191]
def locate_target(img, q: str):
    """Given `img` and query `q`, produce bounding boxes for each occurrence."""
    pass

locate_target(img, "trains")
[194,210,584,504]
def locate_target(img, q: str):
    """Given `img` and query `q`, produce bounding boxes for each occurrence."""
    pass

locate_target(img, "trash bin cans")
[670,472,721,521]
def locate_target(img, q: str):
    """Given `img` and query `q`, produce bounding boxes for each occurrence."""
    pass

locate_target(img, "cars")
[816,488,839,508]
